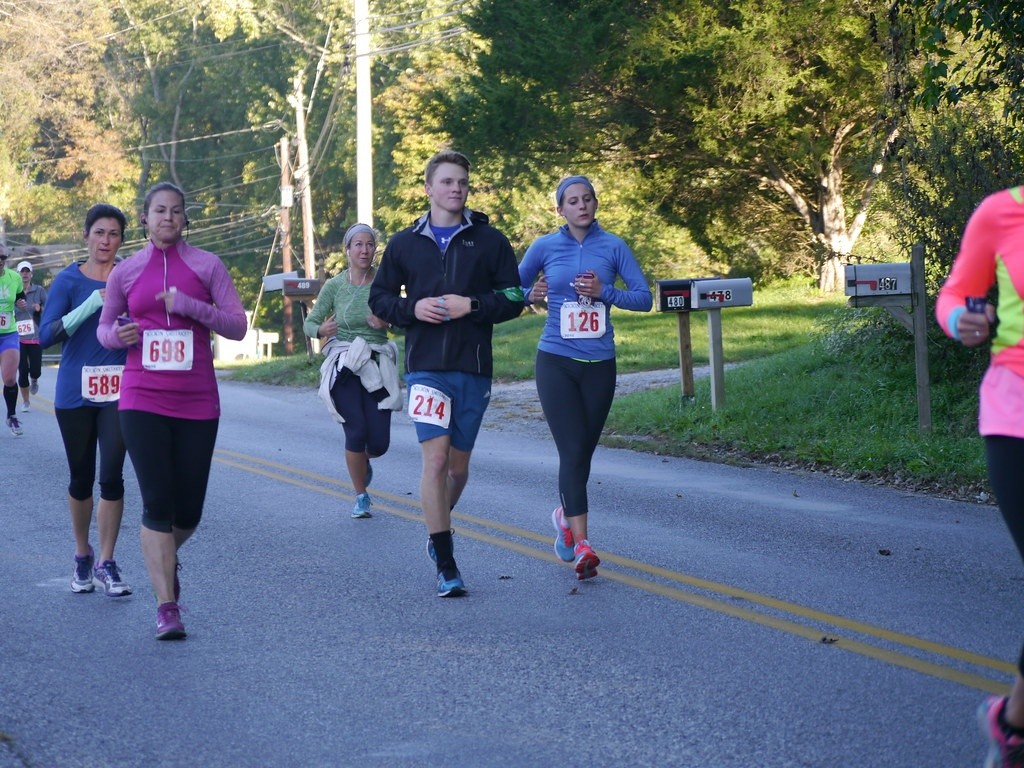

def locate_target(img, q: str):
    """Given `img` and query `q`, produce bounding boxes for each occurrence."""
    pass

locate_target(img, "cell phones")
[577,273,596,305]
[116,315,139,349]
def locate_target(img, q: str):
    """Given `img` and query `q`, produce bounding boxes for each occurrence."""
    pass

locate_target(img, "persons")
[0,243,28,435]
[934,185,1024,768]
[96,181,247,642]
[518,174,653,580]
[367,153,524,599]
[39,204,133,596]
[303,223,405,519]
[13,260,47,411]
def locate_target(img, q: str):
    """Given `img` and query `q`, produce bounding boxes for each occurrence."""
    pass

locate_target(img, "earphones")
[570,283,575,287]
[347,250,350,257]
[31,274,33,277]
[141,218,146,224]
[185,218,189,225]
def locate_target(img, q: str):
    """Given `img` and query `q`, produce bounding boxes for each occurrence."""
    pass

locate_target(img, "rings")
[541,291,544,296]
[579,282,585,288]
[975,331,980,337]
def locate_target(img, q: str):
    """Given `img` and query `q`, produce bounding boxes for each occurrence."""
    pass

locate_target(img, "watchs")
[469,296,480,313]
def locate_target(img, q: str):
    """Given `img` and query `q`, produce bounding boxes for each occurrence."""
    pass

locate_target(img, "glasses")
[0,255,7,260]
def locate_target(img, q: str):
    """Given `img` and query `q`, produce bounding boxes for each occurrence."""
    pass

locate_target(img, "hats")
[17,261,33,272]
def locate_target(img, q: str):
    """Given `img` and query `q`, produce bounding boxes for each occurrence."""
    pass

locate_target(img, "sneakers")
[350,492,373,518]
[978,695,1024,768]
[426,537,436,564]
[174,554,183,602]
[71,545,95,593]
[22,400,30,411]
[156,600,186,638]
[436,558,466,597]
[366,459,372,486]
[552,506,574,562]
[92,559,132,597]
[572,533,600,580]
[30,378,38,394]
[5,413,21,436]
